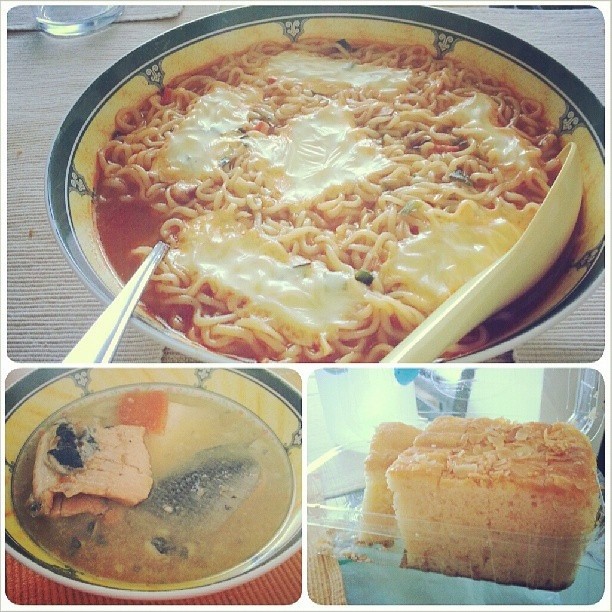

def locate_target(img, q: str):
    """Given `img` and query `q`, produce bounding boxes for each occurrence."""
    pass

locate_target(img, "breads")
[386,415,598,589]
[359,421,423,545]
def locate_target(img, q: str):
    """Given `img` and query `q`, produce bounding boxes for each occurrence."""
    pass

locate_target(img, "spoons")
[374,139,585,364]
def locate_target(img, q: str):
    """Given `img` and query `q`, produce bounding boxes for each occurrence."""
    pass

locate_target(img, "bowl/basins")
[7,368,302,600]
[44,5,604,368]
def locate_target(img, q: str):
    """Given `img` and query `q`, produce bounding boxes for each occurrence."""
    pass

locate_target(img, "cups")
[35,5,127,36]
